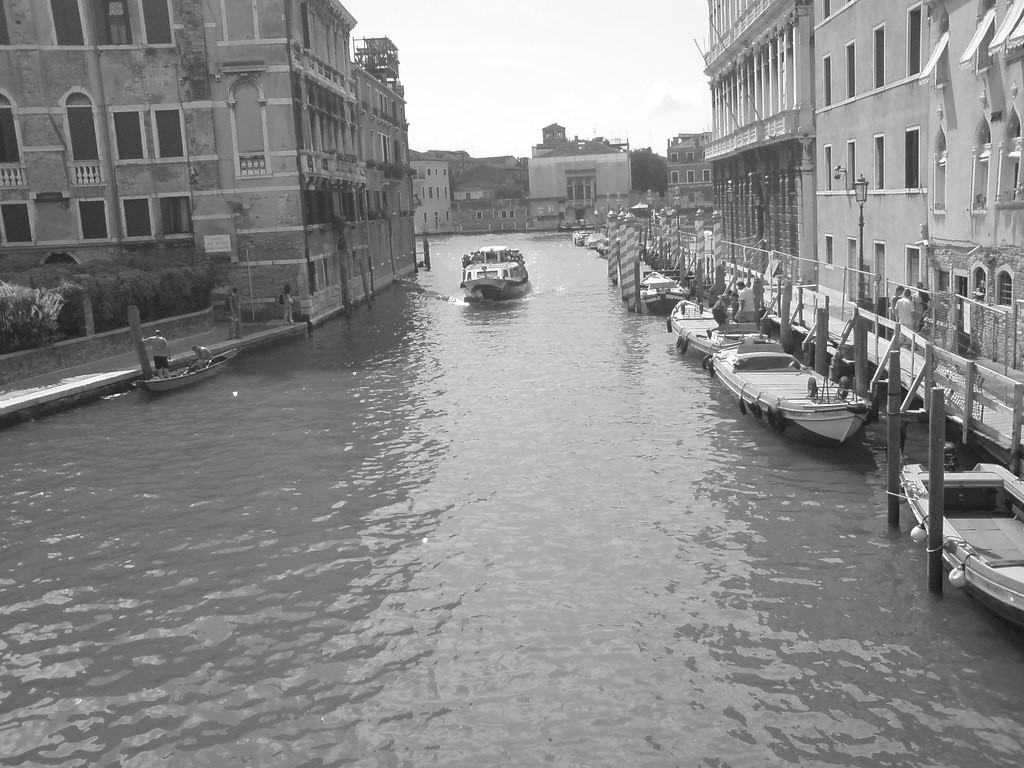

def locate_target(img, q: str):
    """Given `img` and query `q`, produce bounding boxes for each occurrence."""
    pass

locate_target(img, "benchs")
[919,472,1004,489]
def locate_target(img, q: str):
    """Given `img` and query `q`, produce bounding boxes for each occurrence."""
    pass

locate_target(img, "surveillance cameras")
[834,165,841,171]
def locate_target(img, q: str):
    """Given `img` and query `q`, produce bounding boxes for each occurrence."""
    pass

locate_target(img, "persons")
[706,291,738,339]
[192,345,213,369]
[141,330,171,379]
[283,285,295,325]
[734,281,756,323]
[227,288,242,340]
[461,251,481,268]
[892,282,930,350]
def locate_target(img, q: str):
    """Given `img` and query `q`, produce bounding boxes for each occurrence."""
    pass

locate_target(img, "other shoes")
[229,337,232,340]
[235,335,242,338]
[706,330,711,339]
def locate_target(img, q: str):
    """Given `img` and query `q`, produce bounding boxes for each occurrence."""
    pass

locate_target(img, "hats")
[232,287,239,292]
[284,285,291,292]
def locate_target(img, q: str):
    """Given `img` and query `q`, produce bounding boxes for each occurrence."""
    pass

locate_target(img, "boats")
[573,230,690,316]
[143,345,243,393]
[899,462,1024,631]
[460,246,529,304]
[665,299,779,362]
[709,344,873,451]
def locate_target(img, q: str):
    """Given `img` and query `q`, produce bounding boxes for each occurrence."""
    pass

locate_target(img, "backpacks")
[279,293,285,305]
[224,295,239,312]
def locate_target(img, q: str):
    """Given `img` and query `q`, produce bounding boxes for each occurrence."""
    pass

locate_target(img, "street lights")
[674,186,682,259]
[594,210,598,234]
[646,188,653,239]
[850,173,868,308]
[727,178,734,274]
[606,192,611,213]
[615,192,623,212]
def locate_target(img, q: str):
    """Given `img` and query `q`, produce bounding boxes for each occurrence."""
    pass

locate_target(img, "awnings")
[987,0,1024,55]
[959,9,995,70]
[919,33,949,85]
